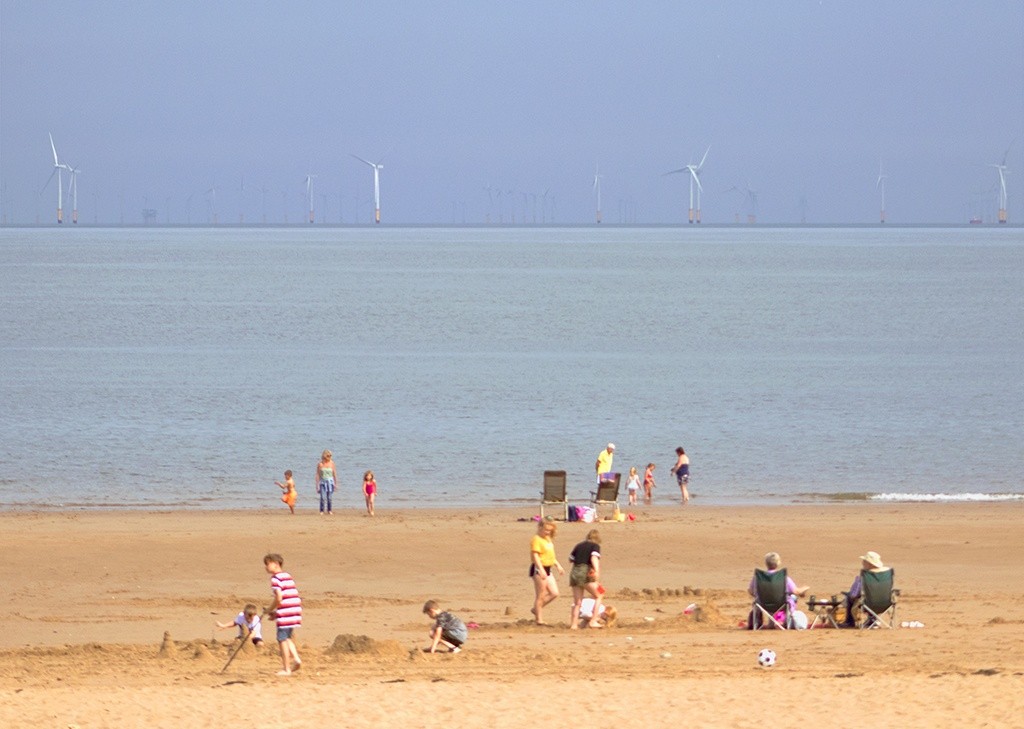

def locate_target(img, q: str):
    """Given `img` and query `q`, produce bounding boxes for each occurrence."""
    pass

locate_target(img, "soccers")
[758,649,777,668]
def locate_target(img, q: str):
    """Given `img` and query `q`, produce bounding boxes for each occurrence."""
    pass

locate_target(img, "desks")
[806,600,845,629]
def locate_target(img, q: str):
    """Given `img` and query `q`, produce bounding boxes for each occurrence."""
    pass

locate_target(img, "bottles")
[808,594,817,612]
[831,593,839,611]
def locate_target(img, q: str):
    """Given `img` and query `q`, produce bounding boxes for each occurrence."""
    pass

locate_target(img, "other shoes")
[453,647,461,652]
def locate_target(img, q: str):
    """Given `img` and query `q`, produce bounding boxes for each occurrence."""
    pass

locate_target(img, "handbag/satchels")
[576,506,584,518]
[851,596,868,625]
[569,505,577,520]
[581,506,594,523]
[792,611,809,630]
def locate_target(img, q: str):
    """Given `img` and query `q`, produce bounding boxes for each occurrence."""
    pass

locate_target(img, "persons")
[529,522,565,625]
[568,529,616,630]
[274,470,297,514]
[423,600,467,654]
[316,450,336,515]
[838,551,883,629]
[362,472,377,516]
[262,554,303,675]
[215,604,264,648]
[748,552,810,628]
[644,463,656,498]
[625,467,642,505]
[671,447,690,504]
[595,443,614,485]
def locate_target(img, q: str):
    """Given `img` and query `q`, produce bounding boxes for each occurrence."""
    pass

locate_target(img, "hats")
[860,551,884,568]
[608,442,615,448]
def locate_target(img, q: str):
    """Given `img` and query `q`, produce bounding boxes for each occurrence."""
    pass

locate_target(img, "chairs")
[858,568,901,630]
[753,567,800,629]
[589,472,623,519]
[540,470,569,519]
